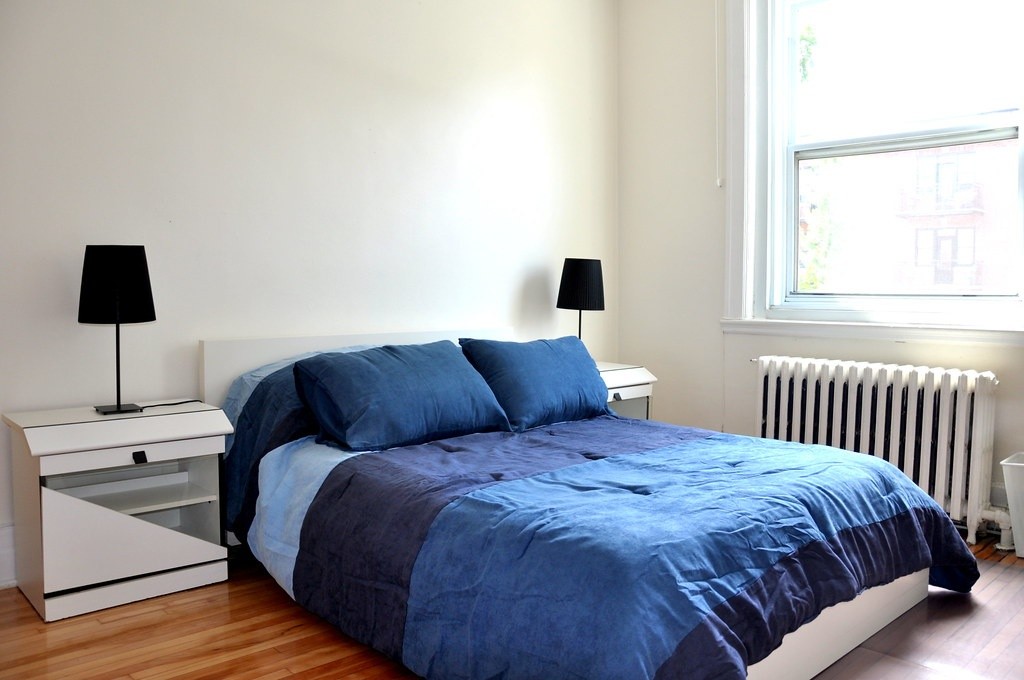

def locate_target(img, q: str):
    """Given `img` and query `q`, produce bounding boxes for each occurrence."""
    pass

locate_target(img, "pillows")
[459,336,616,434]
[292,340,514,451]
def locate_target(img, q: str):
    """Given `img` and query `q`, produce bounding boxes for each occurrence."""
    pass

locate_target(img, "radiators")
[745,354,1019,552]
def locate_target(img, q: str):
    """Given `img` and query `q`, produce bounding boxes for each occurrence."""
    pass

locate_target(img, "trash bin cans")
[999,451,1024,559]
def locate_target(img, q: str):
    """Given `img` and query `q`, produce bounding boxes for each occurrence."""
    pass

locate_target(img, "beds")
[197,334,981,680]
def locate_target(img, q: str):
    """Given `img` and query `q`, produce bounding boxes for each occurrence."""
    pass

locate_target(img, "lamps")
[77,244,157,414]
[556,257,606,339]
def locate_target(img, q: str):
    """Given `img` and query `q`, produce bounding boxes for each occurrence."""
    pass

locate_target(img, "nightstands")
[1,398,235,624]
[592,361,657,422]
